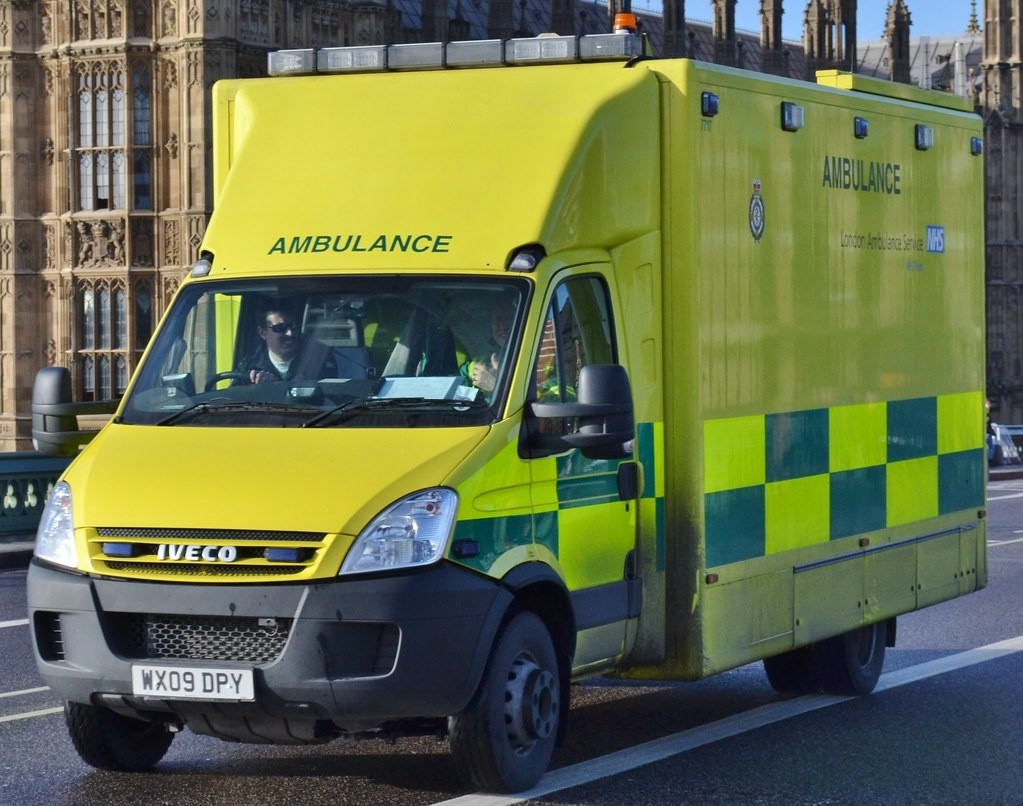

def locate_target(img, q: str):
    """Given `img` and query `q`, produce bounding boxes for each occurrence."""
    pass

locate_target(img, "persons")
[985,400,996,467]
[451,296,577,403]
[229,301,338,388]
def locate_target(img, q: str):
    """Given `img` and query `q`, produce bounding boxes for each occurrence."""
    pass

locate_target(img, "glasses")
[263,318,299,333]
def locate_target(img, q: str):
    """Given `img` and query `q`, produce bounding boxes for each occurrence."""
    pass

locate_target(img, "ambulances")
[25,12,990,792]
